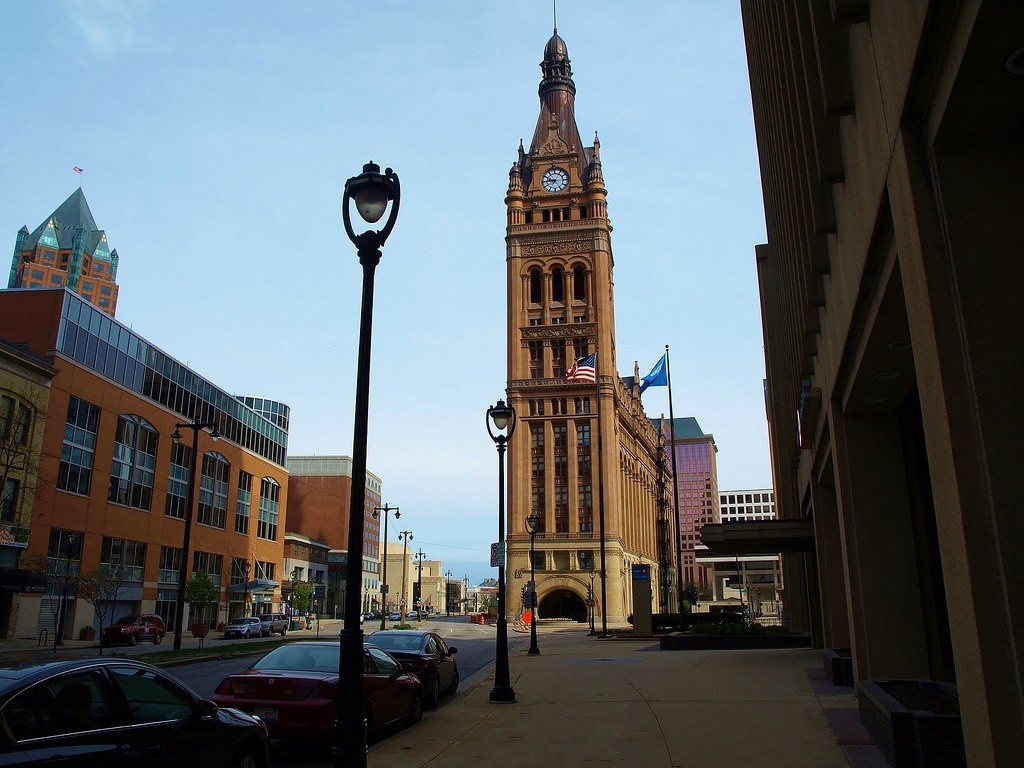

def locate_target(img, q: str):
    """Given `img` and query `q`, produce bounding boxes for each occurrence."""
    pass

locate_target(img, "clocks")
[541,168,568,192]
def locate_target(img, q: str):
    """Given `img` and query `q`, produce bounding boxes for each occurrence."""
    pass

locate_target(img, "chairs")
[296,654,316,670]
[380,637,394,648]
[12,683,93,737]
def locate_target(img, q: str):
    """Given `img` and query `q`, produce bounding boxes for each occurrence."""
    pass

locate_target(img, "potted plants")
[185,566,219,637]
[80,626,96,641]
[287,580,315,629]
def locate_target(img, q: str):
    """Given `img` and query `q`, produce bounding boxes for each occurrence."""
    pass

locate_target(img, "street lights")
[243,563,251,617]
[171,415,223,649]
[486,398,516,703]
[525,513,541,654]
[56,532,77,647]
[290,571,297,628]
[589,570,596,635]
[340,160,402,768]
[396,531,426,625]
[445,570,469,616]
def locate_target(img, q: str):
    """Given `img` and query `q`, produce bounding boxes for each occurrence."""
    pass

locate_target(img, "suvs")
[224,617,261,639]
[102,614,165,646]
[258,614,289,637]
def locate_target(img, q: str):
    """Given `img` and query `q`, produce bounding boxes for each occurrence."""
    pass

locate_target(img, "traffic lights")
[418,594,420,600]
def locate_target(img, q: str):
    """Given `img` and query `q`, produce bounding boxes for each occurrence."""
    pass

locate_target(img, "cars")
[408,611,429,618]
[390,612,401,620]
[213,641,423,759]
[0,660,274,768]
[366,630,459,710]
[359,610,381,625]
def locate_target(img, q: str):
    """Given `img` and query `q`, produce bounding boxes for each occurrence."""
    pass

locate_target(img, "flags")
[72,166,82,174]
[638,353,668,394]
[565,352,596,383]
